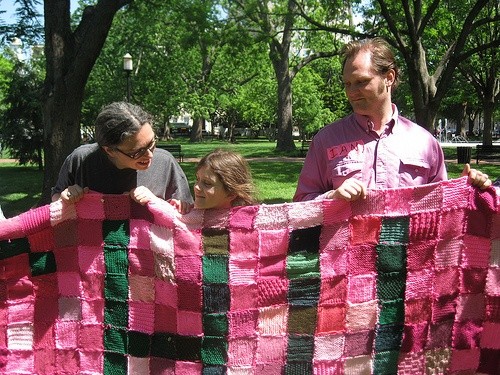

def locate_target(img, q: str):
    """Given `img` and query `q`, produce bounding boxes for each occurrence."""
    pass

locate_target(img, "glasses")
[113,134,158,160]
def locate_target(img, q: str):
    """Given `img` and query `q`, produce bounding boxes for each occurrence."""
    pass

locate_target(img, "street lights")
[122,51,134,104]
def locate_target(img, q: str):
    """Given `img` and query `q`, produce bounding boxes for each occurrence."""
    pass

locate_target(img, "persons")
[169,151,257,214]
[293,38,492,202]
[50,102,195,206]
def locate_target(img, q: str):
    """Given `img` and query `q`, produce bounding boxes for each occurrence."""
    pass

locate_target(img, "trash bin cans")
[456,146,472,165]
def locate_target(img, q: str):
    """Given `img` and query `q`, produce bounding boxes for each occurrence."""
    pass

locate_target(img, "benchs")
[471,145,500,165]
[300,141,311,155]
[157,145,184,163]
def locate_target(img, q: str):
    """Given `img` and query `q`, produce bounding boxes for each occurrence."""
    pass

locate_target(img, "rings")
[67,192,72,196]
